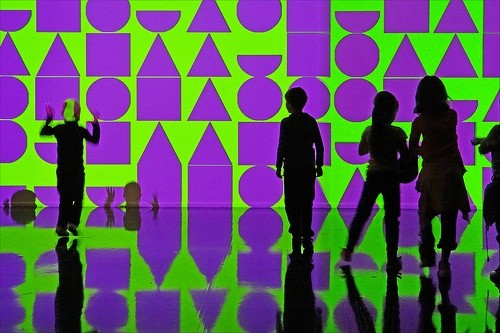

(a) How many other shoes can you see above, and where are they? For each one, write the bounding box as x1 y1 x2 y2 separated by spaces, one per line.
67 224 78 236
438 260 451 270
488 269 500 281
55 225 69 236
419 257 437 267
386 256 404 272
346 244 354 253
288 225 315 238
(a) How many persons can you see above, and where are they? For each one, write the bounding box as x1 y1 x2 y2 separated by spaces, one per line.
333 91 410 271
479 125 500 280
418 278 457 333
104 207 159 230
410 76 471 278
276 88 325 236
276 237 322 333
335 267 403 333
2 189 36 208
4 208 36 224
104 181 160 207
39 97 99 237
